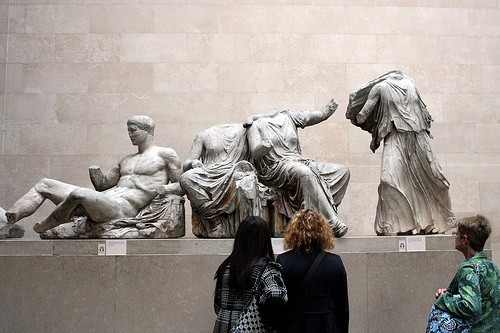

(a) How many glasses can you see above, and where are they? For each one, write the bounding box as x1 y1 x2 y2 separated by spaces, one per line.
452 231 466 238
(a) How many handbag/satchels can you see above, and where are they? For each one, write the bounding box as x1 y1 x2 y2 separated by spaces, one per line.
426 260 482 333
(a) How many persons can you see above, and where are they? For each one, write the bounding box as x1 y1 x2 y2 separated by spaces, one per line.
346 70 459 236
178 122 260 238
274 208 349 333
0 114 186 241
212 215 287 333
435 216 500 333
243 99 350 237
